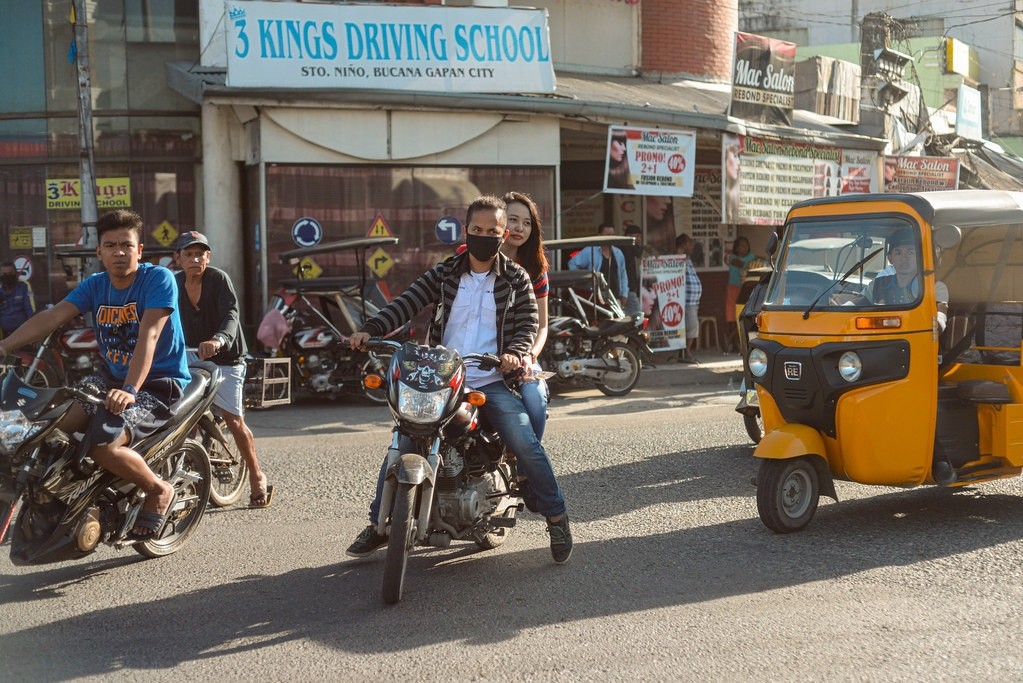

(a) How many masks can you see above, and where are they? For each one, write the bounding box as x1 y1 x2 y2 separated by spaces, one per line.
0 274 18 285
465 225 505 262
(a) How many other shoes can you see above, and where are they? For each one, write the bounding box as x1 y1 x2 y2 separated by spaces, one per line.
934 459 956 485
679 353 701 364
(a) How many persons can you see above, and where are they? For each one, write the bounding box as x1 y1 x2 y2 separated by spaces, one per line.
568 221 629 325
615 225 660 315
725 144 742 224
0 208 192 541
0 259 42 354
646 195 677 255
344 195 573 565
607 129 634 188
842 229 957 487
721 236 756 353
675 233 703 364
173 230 274 510
454 191 547 514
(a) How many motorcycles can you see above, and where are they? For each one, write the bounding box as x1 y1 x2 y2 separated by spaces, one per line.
336 332 527 603
243 284 404 412
0 297 254 557
537 296 657 397
736 190 1023 535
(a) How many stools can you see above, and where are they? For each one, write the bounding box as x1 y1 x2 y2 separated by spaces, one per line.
696 317 720 351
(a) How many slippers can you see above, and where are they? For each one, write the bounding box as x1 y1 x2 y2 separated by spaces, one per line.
248 484 274 509
129 491 177 541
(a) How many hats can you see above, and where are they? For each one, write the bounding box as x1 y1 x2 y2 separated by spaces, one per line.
176 231 211 252
888 228 915 253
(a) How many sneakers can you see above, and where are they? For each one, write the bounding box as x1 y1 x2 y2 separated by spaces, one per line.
543 512 573 564
516 479 539 512
345 520 391 557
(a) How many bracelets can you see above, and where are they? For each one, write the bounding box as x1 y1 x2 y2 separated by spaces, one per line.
122 384 138 398
529 352 537 364
212 335 226 347
